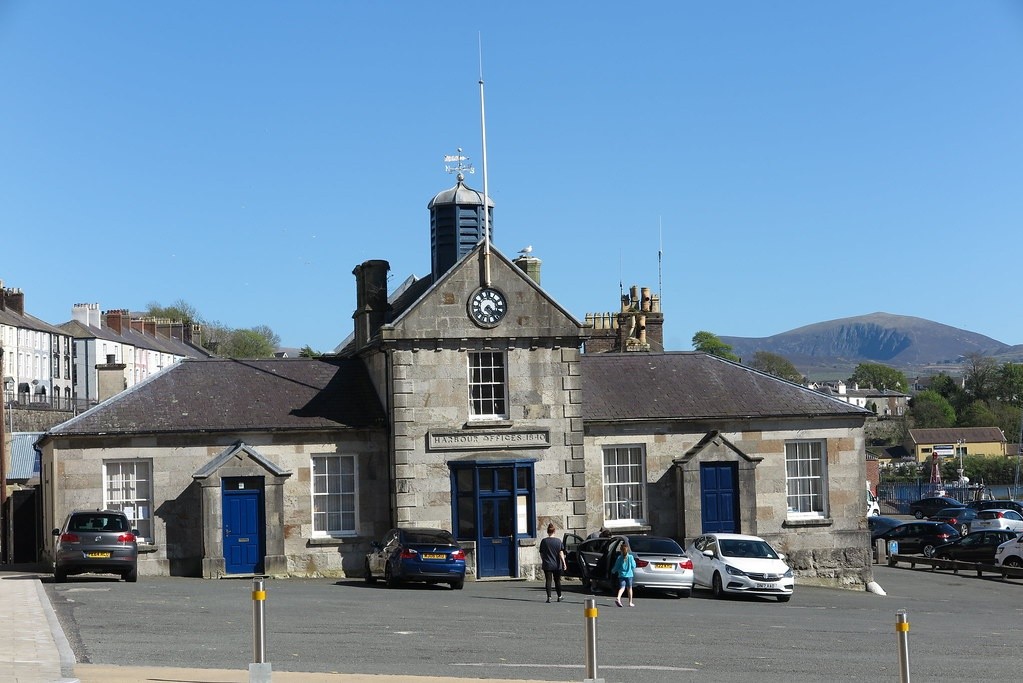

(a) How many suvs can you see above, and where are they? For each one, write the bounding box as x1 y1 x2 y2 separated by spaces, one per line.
994 533 1023 578
52 507 140 583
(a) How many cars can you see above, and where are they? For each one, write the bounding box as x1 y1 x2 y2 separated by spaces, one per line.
686 534 795 602
910 498 969 519
363 527 466 591
928 507 976 537
867 489 881 518
560 532 695 598
874 522 961 558
966 498 1023 516
972 509 1023 533
868 517 903 543
930 530 1017 569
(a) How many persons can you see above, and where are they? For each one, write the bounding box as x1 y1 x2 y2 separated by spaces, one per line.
612 543 636 607
539 523 567 603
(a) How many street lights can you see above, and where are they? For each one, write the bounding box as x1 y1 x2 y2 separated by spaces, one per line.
957 438 966 483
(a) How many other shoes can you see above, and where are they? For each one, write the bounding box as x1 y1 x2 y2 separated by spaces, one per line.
615 598 623 607
546 598 551 603
629 603 635 607
558 596 565 602
